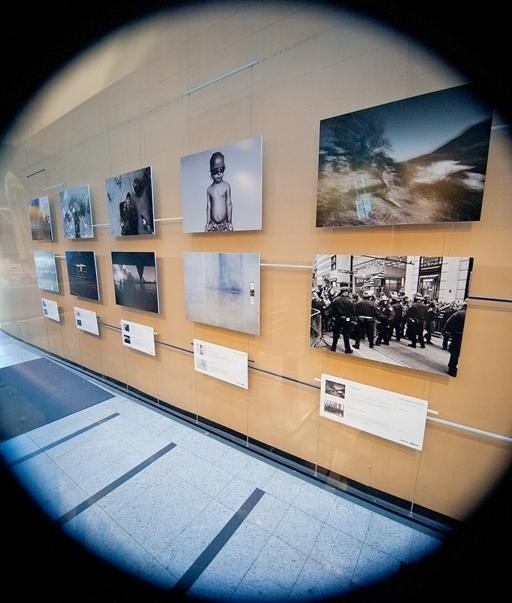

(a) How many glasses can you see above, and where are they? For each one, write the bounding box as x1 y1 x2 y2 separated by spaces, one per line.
213 166 225 175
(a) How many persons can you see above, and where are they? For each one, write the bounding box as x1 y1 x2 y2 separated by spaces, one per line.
204 152 234 232
311 279 468 376
120 192 135 230
